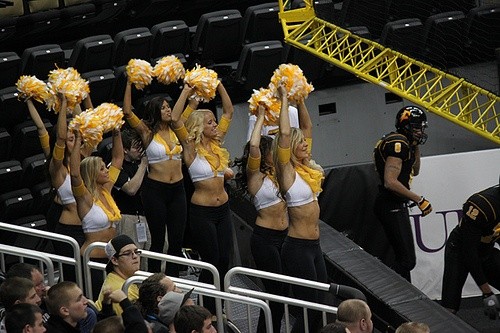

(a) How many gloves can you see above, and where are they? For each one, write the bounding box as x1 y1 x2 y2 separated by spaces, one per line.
482 292 497 316
418 196 433 217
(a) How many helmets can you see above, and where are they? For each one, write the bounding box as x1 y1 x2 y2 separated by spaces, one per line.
395 106 429 145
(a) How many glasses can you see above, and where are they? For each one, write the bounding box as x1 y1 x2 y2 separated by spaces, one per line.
117 250 142 258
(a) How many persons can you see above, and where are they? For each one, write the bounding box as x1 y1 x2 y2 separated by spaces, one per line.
373 105 432 284
438 182 499 320
170 67 234 328
105 127 150 274
335 299 373 333
319 322 352 333
395 321 430 333
122 58 201 282
70 120 123 301
0 233 195 333
25 87 87 296
277 80 330 333
174 305 218 333
236 101 290 333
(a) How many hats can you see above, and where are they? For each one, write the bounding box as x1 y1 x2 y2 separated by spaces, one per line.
104 234 136 274
157 288 194 325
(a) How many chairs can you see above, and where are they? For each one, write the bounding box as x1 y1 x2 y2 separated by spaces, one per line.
0 0 500 261
